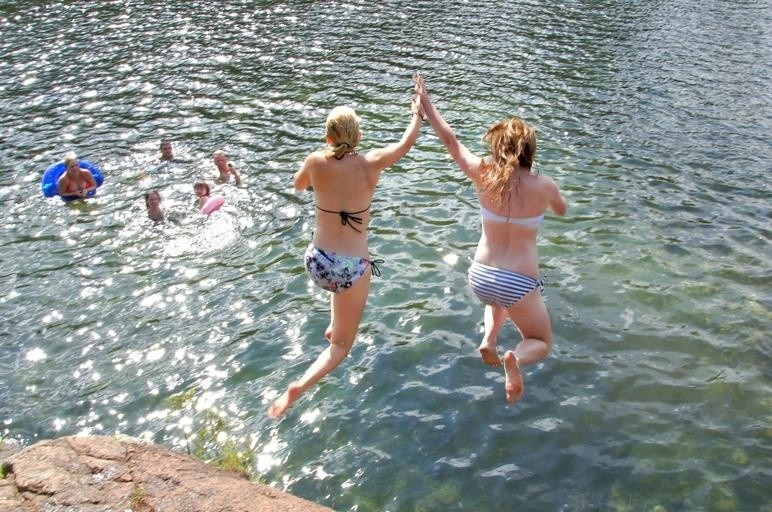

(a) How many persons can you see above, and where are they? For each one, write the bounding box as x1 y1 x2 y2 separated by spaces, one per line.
56 155 97 199
412 74 566 403
144 190 165 221
194 181 210 209
213 148 241 185
159 140 174 161
269 93 424 418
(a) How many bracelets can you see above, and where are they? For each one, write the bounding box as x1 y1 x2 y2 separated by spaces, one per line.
413 109 424 121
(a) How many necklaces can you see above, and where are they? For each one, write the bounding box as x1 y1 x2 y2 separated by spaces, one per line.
329 147 358 156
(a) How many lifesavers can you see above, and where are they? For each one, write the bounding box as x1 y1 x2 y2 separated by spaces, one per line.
201 195 224 214
42 160 104 200
